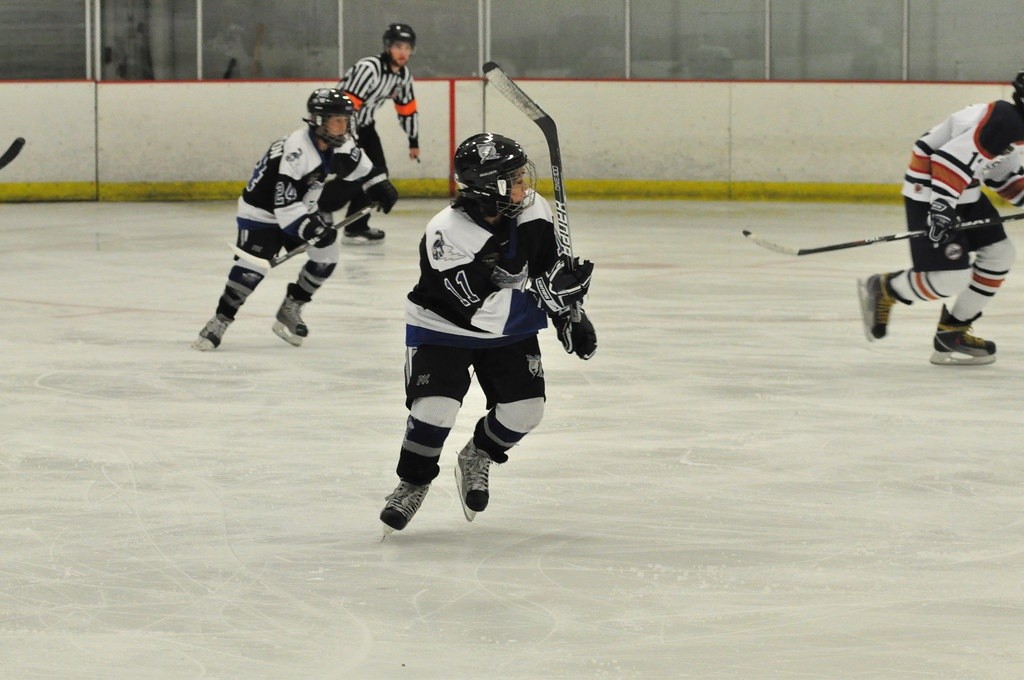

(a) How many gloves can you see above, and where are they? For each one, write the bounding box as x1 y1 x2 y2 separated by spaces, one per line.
928 199 963 244
551 305 597 359
534 256 593 317
298 213 336 249
366 179 400 214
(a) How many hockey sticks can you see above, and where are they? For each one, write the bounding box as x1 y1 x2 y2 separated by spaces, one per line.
482 61 585 351
268 197 385 267
0 137 27 174
742 212 1024 257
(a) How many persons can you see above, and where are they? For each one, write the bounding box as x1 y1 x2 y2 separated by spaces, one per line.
857 69 1024 366
192 87 399 350
379 131 597 542
319 23 421 244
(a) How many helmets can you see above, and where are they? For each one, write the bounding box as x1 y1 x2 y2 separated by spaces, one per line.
382 22 417 49
1012 71 1023 116
452 133 536 219
303 87 356 146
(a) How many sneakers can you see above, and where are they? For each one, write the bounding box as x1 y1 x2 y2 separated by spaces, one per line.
380 468 435 543
454 438 492 523
195 304 235 350
856 271 913 342
273 283 311 346
928 303 999 364
339 228 385 245
